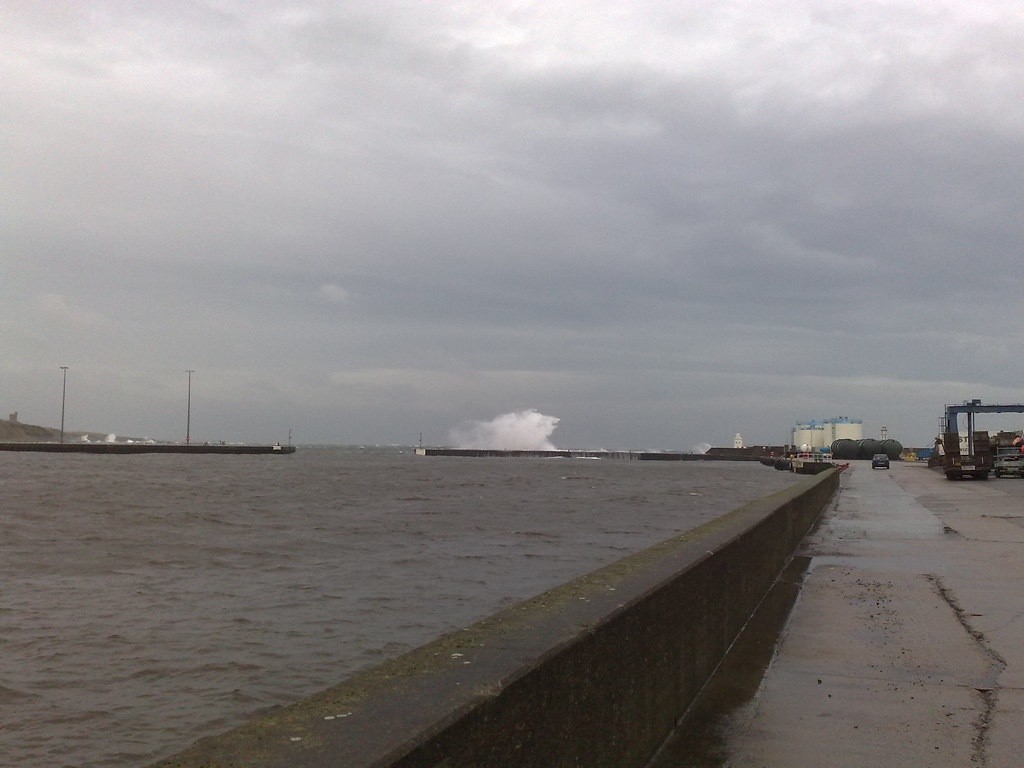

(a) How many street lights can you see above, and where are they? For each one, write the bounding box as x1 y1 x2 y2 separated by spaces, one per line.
59 366 69 444
184 370 196 446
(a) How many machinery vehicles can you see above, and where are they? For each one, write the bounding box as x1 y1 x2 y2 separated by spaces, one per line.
935 399 1024 480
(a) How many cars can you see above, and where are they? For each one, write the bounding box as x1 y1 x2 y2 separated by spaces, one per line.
871 454 890 469
